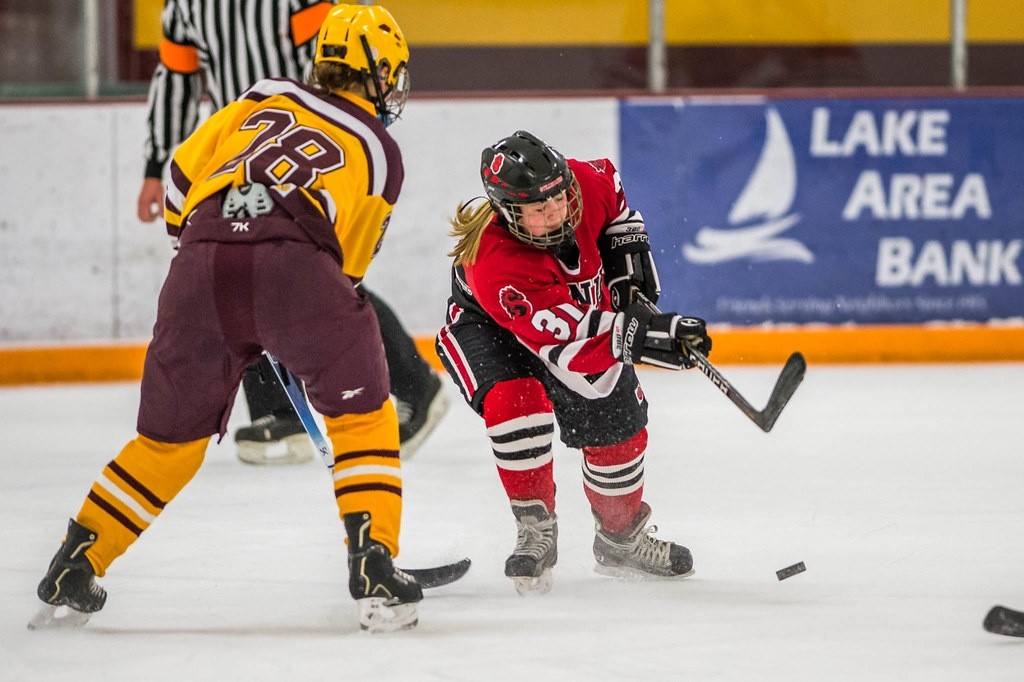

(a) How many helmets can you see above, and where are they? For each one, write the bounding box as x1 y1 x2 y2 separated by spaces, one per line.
480 130 583 249
313 4 410 126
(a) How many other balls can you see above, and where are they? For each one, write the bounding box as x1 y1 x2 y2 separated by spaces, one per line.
776 560 807 582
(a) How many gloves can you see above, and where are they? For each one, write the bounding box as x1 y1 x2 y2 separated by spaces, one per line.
603 232 660 312
621 302 710 371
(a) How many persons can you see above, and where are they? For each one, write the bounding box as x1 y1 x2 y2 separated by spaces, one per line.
435 130 712 595
27 3 424 632
138 0 449 464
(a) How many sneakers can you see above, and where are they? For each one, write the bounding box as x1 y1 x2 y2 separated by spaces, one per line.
504 482 559 598
342 510 424 635
590 501 694 581
27 518 107 630
236 412 315 465
396 372 447 459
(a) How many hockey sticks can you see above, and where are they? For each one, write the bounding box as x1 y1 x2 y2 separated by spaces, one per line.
263 353 473 589
629 286 806 434
